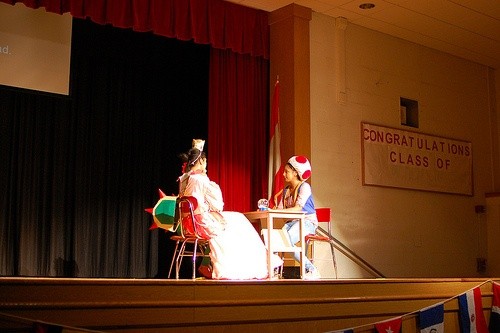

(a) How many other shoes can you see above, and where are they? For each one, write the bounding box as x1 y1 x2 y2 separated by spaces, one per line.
303 267 321 280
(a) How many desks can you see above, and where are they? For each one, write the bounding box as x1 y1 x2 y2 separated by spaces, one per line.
244 212 307 280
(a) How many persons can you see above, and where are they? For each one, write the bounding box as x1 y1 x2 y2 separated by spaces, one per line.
268 155 323 279
177 139 284 280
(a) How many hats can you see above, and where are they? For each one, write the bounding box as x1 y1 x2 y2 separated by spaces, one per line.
288 155 312 181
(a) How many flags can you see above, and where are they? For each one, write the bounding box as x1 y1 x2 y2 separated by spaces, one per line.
374 318 402 333
458 287 488 333
333 329 353 333
419 304 445 333
488 280 500 333
267 75 286 211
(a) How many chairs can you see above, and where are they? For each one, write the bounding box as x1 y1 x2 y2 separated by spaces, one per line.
279 207 338 278
167 196 212 280
